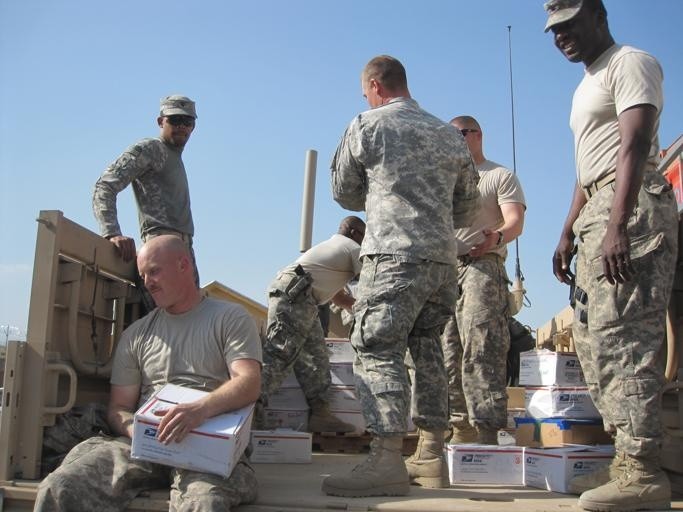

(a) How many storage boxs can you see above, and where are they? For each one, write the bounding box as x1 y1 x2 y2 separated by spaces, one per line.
265 338 371 434
515 350 618 498
443 442 523 487
131 383 252 479
250 430 312 463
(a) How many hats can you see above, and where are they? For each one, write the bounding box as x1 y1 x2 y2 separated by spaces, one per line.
160 96 197 118
543 1 584 33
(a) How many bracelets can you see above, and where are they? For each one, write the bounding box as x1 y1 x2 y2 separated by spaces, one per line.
496 230 503 245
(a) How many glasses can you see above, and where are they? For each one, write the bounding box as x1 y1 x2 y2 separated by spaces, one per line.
461 129 478 137
166 117 194 126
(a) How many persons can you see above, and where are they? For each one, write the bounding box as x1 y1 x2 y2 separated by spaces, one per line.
251 216 366 434
32 235 263 512
92 96 198 312
445 115 525 444
543 0 678 512
319 54 481 499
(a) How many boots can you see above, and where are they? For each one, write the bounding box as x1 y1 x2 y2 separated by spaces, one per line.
579 457 671 510
404 429 450 488
567 452 628 494
322 436 410 497
307 399 355 432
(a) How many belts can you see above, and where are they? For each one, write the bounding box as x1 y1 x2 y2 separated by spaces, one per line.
459 253 504 266
582 165 653 199
